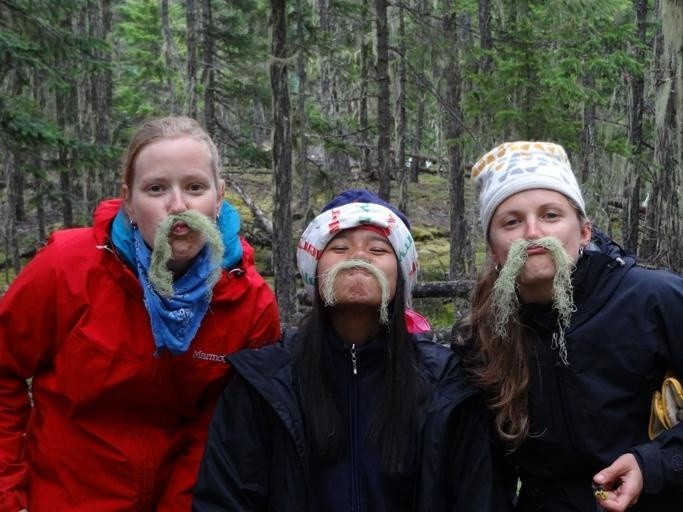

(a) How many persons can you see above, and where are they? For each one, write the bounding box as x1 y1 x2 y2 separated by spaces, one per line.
193 187 497 511
452 138 682 510
0 115 284 511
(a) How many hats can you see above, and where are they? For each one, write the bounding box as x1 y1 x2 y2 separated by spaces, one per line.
471 141 587 242
295 189 419 306
